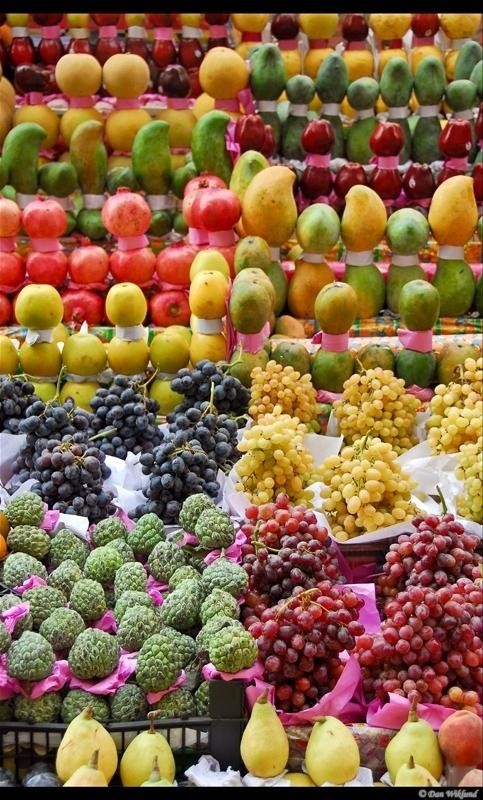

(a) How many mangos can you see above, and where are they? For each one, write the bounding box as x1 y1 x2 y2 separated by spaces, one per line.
249 40 482 163
0 109 233 239
229 150 483 318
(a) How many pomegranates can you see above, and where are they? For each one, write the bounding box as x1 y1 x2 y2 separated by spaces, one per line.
1 170 242 327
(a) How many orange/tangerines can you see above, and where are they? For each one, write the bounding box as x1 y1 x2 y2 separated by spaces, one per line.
229 235 483 392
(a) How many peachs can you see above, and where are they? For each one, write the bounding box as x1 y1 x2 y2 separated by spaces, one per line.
437 710 483 768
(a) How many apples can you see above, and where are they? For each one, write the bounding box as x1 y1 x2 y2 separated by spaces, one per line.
236 101 483 218
1 248 229 416
1 13 229 99
272 14 441 39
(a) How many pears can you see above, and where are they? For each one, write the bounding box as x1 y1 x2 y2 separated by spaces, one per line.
55 687 444 787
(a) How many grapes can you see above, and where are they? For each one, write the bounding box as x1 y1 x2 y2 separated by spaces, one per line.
0 358 252 527
242 492 483 712
235 356 483 542
